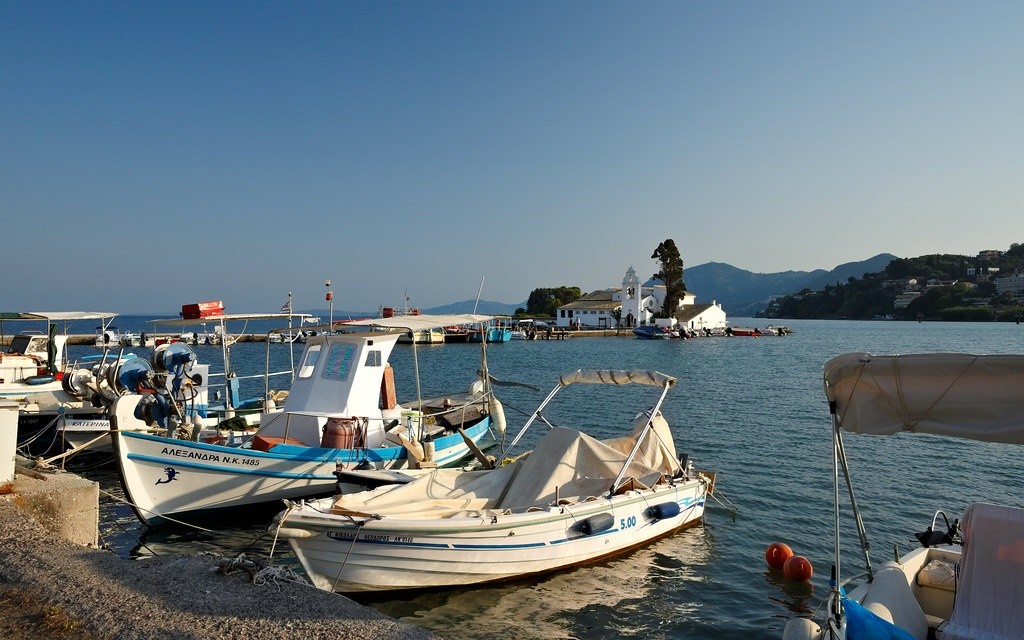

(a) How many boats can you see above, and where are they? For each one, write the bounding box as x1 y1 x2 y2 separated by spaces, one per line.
296 330 326 343
632 325 671 340
94 326 119 347
208 326 235 345
731 327 763 336
821 351 1024 640
56 314 313 451
180 331 206 345
331 314 538 344
0 311 139 439
266 335 292 343
109 313 496 527
269 371 716 593
124 333 141 346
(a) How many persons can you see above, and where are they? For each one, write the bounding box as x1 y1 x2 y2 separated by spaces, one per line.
569 317 572 325
575 316 580 331
